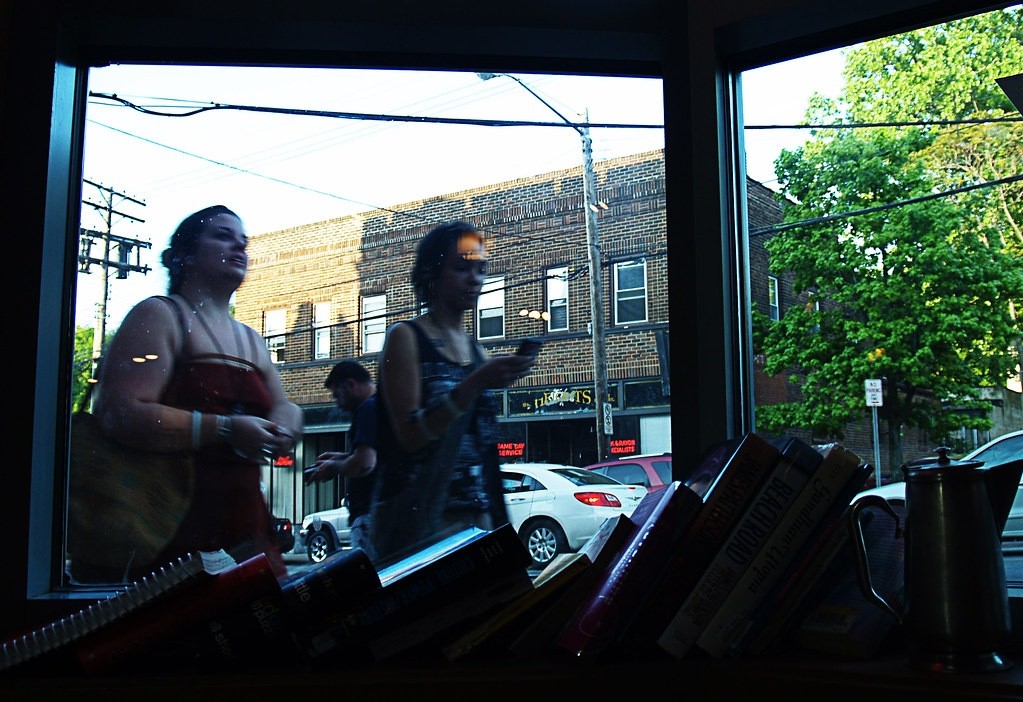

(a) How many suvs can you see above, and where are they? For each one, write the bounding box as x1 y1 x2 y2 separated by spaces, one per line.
577 452 672 493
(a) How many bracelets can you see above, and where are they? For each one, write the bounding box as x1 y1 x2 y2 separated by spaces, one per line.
216 415 231 446
191 410 202 450
443 393 468 420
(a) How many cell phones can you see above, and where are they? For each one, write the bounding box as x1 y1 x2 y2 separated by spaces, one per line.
517 338 544 357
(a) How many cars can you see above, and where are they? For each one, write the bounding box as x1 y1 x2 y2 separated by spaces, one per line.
469 464 648 569
850 430 1023 605
275 517 296 555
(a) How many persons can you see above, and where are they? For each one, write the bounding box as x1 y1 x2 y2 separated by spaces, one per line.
306 361 375 571
78 204 303 582
376 222 538 553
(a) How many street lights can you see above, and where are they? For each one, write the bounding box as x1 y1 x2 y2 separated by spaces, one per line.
479 70 613 465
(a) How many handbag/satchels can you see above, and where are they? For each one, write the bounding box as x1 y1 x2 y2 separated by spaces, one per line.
64 297 194 568
